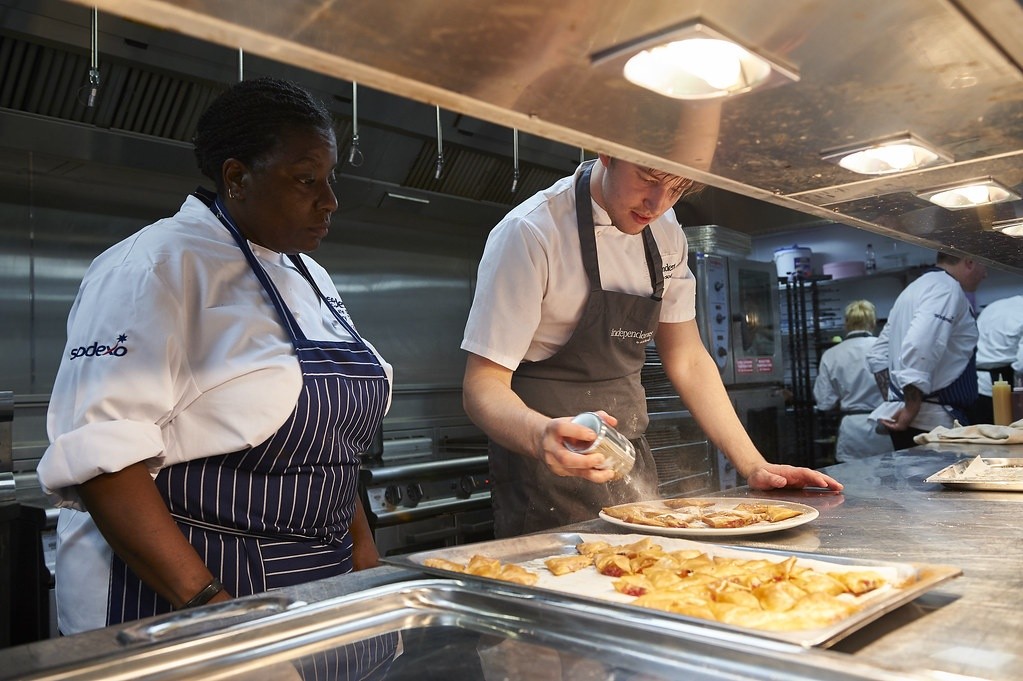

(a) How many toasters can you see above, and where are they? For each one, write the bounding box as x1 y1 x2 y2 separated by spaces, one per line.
865 243 876 274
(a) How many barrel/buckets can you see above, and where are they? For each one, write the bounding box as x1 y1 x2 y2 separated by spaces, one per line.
772 243 813 287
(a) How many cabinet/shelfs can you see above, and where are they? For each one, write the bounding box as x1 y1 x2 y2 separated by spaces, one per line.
778 264 928 465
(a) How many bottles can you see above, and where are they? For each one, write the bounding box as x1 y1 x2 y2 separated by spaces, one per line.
992 374 1023 426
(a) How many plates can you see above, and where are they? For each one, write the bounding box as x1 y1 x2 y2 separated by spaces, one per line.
598 497 819 535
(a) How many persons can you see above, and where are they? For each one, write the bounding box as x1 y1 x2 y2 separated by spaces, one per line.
811 298 898 465
973 294 1023 425
460 155 843 540
870 249 980 453
35 74 394 636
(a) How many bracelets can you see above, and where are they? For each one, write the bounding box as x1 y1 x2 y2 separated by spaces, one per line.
186 577 223 610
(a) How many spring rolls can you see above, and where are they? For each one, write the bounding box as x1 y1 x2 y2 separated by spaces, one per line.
423 537 886 635
600 497 805 529
